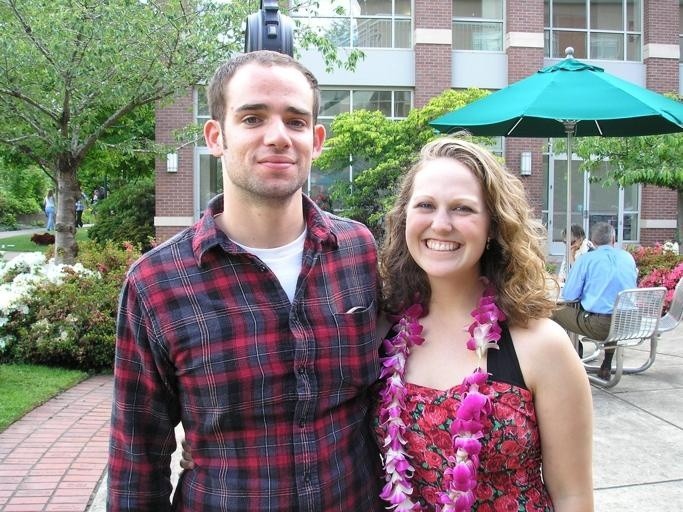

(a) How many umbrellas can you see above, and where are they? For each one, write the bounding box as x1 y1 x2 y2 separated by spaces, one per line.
427 47 683 280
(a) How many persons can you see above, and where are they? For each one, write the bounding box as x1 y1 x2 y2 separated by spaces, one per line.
180 137 594 512
548 222 638 381
108 50 387 512
43 187 101 231
557 224 595 287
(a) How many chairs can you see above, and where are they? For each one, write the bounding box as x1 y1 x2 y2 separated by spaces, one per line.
582 275 683 391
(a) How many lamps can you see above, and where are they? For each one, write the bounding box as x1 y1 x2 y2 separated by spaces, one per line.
519 151 532 178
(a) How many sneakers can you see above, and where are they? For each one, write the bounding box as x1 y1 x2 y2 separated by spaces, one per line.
597 369 611 379
